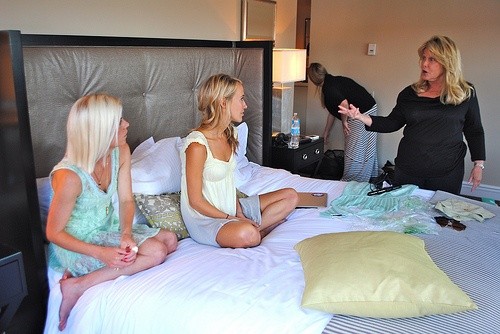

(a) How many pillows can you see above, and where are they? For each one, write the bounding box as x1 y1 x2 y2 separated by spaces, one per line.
293 231 478 319
35 122 251 240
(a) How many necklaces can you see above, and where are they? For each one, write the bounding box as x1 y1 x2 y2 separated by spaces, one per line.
94 167 104 185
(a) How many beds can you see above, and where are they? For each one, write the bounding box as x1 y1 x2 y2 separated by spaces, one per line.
42 163 500 334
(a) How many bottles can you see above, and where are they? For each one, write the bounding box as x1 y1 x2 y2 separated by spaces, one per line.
290 113 300 146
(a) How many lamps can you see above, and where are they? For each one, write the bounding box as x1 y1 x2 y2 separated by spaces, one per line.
272 48 307 90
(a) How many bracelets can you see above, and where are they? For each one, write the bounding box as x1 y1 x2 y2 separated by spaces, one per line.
474 163 484 169
226 214 229 219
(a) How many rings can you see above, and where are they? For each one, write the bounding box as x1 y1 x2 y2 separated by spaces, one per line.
131 246 138 253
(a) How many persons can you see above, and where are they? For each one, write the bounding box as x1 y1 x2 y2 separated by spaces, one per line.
45 94 177 331
179 73 298 248
336 34 485 195
308 62 379 182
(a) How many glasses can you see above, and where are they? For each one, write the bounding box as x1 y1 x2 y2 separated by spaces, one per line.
434 216 466 231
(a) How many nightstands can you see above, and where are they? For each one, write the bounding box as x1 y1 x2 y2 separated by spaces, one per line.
272 135 324 174
0 243 29 334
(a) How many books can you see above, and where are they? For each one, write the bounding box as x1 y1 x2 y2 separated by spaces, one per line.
297 192 328 208
